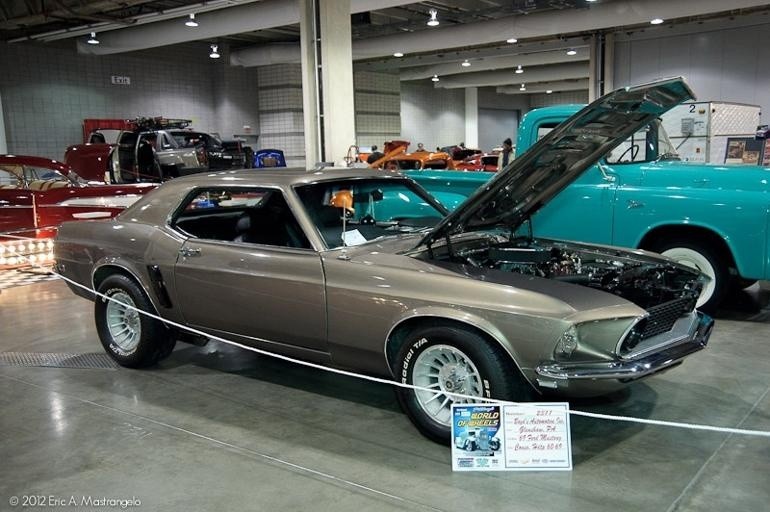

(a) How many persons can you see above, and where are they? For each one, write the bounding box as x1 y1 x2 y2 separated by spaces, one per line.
497 138 516 172
368 145 385 164
416 143 425 152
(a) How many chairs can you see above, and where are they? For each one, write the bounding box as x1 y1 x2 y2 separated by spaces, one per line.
231 206 284 245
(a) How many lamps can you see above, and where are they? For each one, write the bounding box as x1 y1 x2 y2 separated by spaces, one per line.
87 31 100 45
425 7 440 27
514 65 524 74
431 74 439 82
186 13 199 28
519 83 527 91
207 44 221 59
